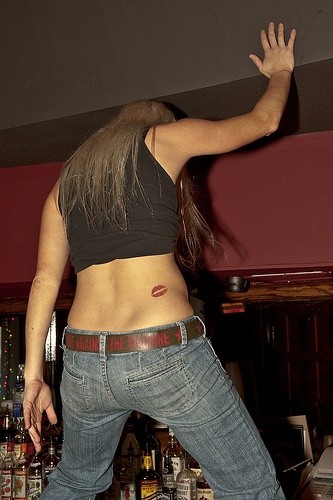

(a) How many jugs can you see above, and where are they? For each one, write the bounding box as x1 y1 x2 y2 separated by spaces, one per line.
226 276 247 292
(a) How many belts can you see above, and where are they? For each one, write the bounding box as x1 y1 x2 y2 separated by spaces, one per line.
62 319 204 353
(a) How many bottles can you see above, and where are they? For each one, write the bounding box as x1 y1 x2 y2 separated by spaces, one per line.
0 366 65 500
96 412 215 500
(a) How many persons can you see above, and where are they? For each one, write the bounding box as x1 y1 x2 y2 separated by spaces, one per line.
22 21 297 500
174 240 246 402
